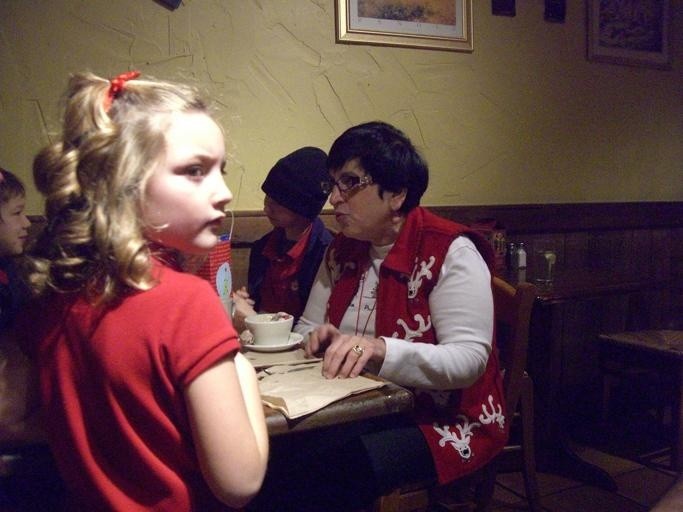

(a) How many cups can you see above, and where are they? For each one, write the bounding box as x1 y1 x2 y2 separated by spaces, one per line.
246 312 294 346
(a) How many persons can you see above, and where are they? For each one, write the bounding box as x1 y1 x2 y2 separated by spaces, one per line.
0 168 32 318
0 68 271 510
234 145 334 322
293 120 509 512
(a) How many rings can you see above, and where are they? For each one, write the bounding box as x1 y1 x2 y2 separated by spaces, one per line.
351 343 364 356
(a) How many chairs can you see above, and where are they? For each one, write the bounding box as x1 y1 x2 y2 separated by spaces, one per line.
370 260 554 510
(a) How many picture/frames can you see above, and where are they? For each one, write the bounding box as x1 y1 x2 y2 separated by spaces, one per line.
585 1 674 68
333 1 474 56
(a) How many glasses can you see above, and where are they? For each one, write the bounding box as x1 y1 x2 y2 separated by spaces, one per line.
320 173 374 194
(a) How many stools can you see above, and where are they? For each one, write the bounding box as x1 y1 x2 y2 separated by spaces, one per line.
599 319 683 471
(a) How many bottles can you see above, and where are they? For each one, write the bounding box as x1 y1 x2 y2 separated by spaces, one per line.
486 227 530 273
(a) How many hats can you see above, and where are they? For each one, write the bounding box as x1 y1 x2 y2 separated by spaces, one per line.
261 147 332 217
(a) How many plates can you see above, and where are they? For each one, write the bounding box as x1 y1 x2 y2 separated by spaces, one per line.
239 331 304 352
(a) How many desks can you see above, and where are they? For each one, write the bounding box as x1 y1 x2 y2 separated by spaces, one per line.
488 265 678 490
258 342 413 438
